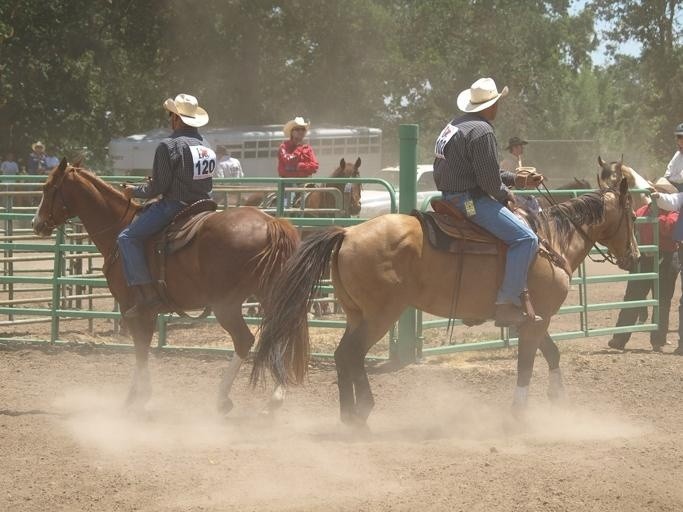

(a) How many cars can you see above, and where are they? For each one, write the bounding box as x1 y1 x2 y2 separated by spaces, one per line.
350 163 442 219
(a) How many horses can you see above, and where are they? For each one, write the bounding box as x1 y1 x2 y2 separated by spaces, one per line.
248 176 642 432
597 154 683 355
30 154 311 415
235 156 364 319
534 171 593 208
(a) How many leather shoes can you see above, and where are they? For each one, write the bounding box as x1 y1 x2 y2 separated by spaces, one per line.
495 303 543 327
125 299 161 319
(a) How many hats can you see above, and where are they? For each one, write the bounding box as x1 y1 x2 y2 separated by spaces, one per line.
505 137 529 150
456 75 509 114
31 141 45 152
645 176 680 194
216 145 230 155
162 93 210 127
674 122 683 136
284 117 311 138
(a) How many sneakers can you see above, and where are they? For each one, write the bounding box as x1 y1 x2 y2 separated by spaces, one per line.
608 339 625 350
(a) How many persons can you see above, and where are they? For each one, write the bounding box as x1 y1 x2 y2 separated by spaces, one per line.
606 177 681 353
650 190 683 356
274 117 319 207
0 141 59 206
210 144 242 205
655 123 683 196
116 93 218 320
433 78 543 326
500 136 541 214
